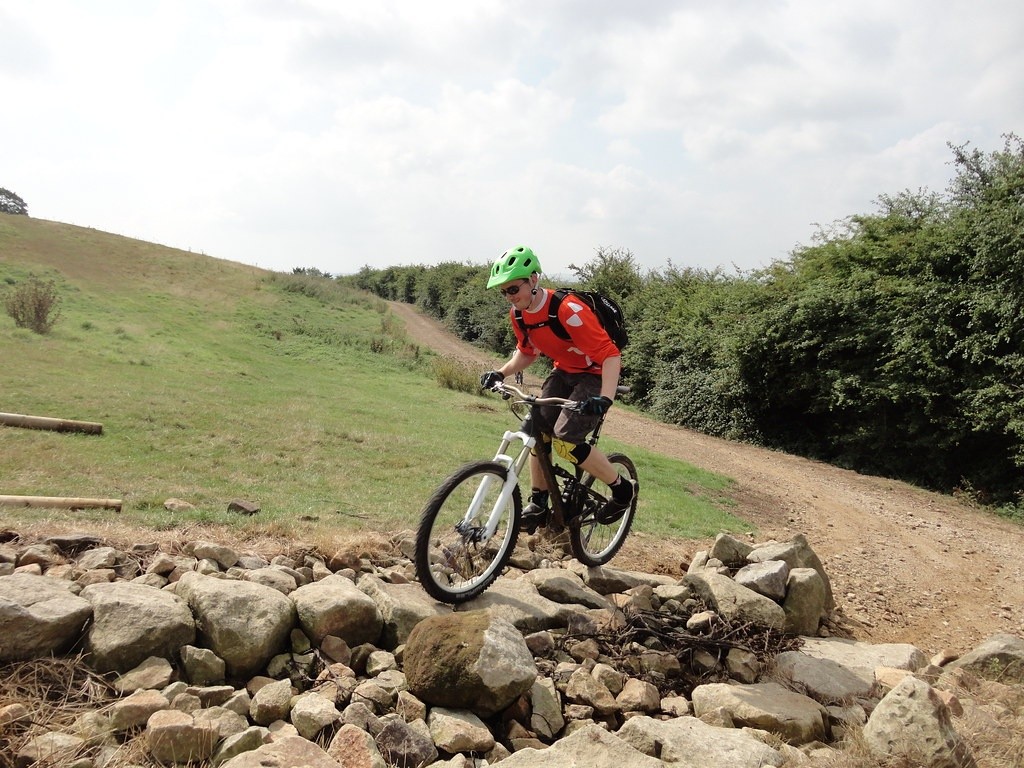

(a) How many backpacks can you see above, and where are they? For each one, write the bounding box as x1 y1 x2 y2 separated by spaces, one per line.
549 285 629 367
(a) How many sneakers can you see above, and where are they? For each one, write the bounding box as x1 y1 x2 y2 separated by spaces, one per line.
596 479 640 525
519 488 551 529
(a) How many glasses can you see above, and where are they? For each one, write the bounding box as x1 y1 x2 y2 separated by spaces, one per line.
500 279 529 297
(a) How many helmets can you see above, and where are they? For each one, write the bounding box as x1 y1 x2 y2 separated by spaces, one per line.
486 245 543 311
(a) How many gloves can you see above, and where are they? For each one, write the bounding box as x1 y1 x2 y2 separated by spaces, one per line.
578 396 613 417
479 370 505 390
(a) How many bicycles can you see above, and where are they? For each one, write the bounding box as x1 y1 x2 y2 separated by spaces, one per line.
413 369 640 606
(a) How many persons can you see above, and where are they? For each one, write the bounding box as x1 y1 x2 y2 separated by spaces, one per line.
481 245 640 525
